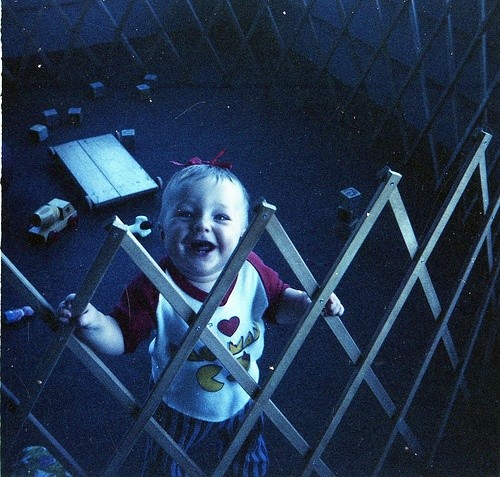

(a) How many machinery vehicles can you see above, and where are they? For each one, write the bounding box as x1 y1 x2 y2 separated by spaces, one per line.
27 197 81 246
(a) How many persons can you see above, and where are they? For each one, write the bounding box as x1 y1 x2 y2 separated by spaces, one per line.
58 148 344 477
